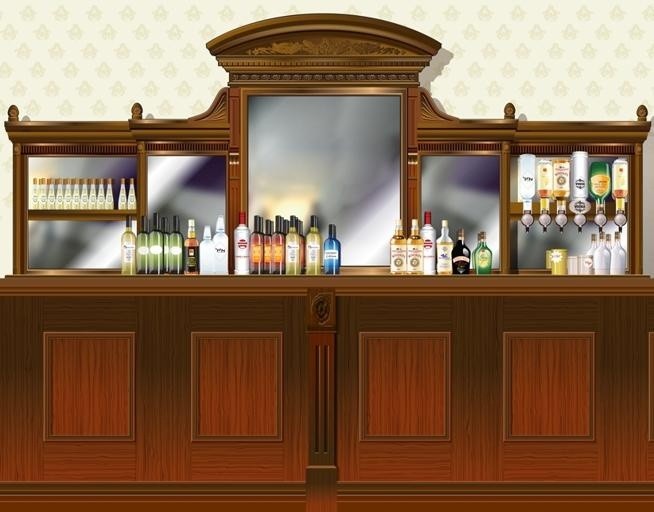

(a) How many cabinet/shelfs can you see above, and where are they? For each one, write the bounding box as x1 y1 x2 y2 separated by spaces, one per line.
0 13 653 511
